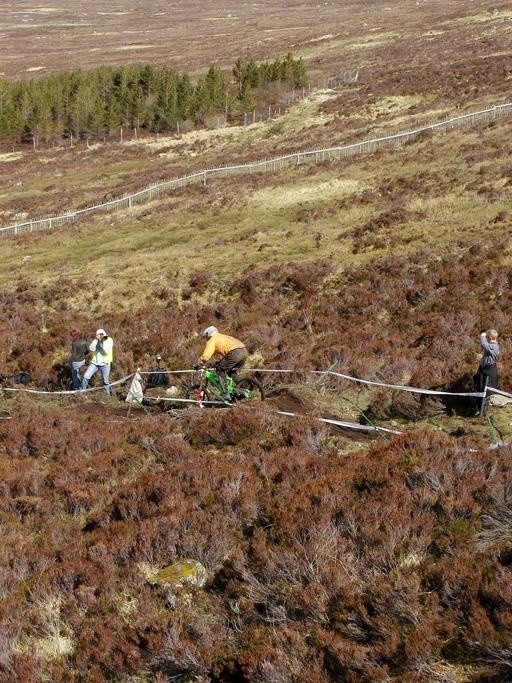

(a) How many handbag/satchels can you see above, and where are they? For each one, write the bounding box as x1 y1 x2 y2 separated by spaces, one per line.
473 372 480 389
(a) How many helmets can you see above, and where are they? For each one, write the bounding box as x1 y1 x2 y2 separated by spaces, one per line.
201 326 218 338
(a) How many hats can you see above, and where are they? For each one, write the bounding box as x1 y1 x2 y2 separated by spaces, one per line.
95 329 106 336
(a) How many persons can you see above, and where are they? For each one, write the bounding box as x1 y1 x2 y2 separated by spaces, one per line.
472 324 499 415
193 324 250 401
69 328 91 390
78 327 115 394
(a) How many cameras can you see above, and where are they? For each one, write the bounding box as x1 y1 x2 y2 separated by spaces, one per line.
99 334 107 339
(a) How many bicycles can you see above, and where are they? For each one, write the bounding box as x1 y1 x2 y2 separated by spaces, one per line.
186 361 265 408
